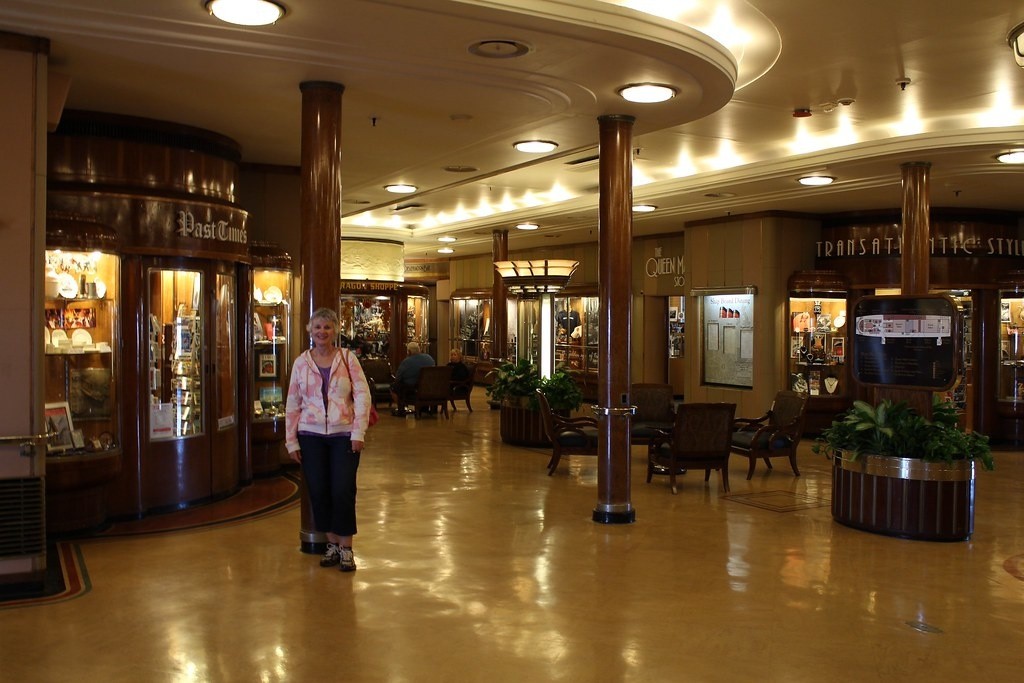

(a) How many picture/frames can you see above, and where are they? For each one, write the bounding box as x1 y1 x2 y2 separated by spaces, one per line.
256 347 281 381
68 367 114 422
259 386 286 412
43 401 76 454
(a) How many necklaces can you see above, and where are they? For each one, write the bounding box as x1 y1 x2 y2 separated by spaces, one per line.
827 378 836 388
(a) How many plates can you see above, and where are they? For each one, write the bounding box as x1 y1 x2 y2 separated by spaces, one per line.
44 327 50 348
71 328 93 347
90 280 107 298
56 273 78 298
51 329 68 348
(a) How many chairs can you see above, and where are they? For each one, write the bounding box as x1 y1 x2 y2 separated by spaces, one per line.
531 383 810 498
357 353 479 420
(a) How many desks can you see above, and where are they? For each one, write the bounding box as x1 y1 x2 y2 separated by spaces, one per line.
45 347 112 424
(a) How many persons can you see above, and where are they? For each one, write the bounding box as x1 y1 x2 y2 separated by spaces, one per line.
388 342 436 419
557 302 582 337
445 347 470 395
282 309 372 573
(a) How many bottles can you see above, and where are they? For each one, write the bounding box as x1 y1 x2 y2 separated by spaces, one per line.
45 263 60 297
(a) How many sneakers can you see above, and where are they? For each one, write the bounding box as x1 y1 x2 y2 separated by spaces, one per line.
338 547 356 570
320 543 343 567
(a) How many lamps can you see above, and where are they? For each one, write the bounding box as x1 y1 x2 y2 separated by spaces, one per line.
995 150 1024 165
797 175 835 186
633 204 659 214
513 139 559 153
383 184 418 194
615 82 679 103
1006 21 1024 70
515 223 541 230
205 0 286 26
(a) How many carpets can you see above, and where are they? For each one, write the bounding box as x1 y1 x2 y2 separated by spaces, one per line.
718 490 833 513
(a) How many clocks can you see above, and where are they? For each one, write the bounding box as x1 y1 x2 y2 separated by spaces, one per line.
98 430 115 450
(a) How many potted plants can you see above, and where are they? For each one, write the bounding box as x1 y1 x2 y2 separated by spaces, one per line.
484 358 584 449
805 392 998 544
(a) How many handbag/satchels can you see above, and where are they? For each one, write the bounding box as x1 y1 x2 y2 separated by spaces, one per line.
338 346 379 426
